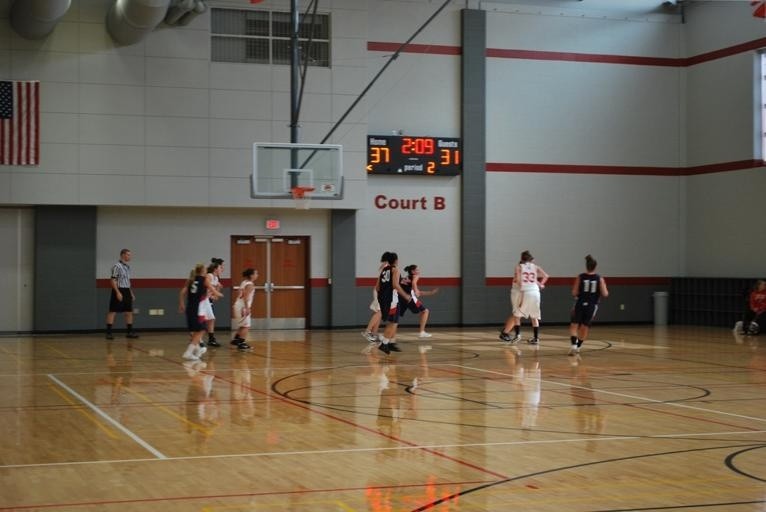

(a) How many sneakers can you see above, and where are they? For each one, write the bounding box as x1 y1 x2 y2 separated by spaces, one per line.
182 333 251 360
511 334 520 344
360 328 402 354
527 337 539 344
105 333 112 339
500 334 510 341
125 332 139 338
575 346 580 353
418 330 432 338
567 344 577 356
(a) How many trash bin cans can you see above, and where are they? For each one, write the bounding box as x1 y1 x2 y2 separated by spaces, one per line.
651 291 671 325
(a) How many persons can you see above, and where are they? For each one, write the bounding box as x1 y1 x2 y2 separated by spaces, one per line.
362 250 440 352
366 347 430 463
500 250 610 354
106 246 140 343
180 257 258 364
104 345 140 429
511 353 611 455
172 366 261 451
739 279 765 336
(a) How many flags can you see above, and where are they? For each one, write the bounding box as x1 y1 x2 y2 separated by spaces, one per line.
2 81 41 166
